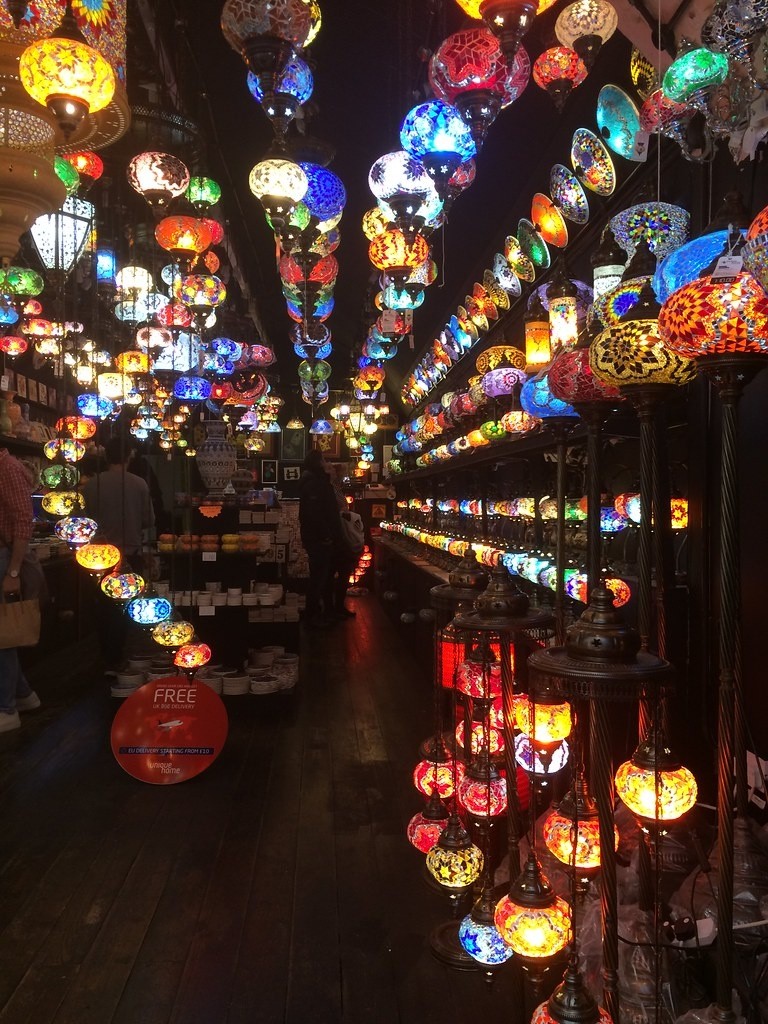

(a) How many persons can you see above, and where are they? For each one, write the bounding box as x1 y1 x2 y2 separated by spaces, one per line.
0 447 41 732
72 444 157 676
302 450 359 630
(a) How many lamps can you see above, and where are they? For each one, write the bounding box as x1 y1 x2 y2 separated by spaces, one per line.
0 0 768 1024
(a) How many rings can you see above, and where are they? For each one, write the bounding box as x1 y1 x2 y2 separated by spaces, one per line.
8 593 14 598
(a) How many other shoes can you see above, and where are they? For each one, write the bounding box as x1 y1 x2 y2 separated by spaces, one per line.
105 669 119 679
304 618 341 631
336 608 356 616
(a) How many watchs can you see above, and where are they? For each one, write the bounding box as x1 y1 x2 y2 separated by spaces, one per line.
5 569 21 578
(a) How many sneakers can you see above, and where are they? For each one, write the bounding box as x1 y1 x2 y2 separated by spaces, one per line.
0 709 21 733
15 692 41 712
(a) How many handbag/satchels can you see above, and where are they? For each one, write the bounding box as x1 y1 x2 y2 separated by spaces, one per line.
0 591 41 650
